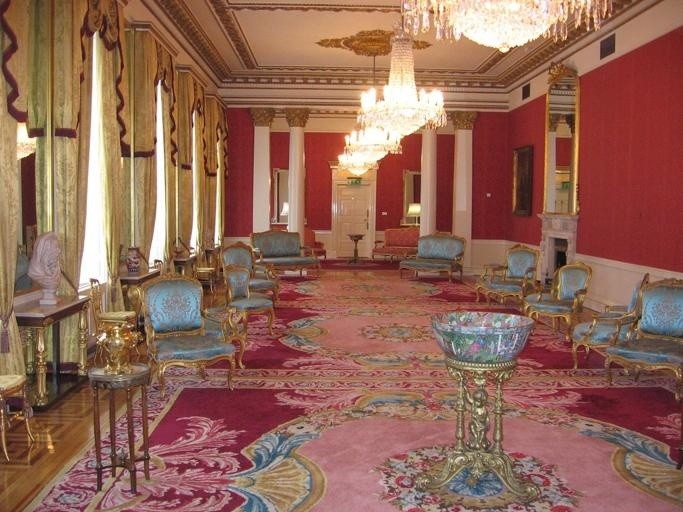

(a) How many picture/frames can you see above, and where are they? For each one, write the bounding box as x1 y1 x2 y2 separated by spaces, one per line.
511 144 531 216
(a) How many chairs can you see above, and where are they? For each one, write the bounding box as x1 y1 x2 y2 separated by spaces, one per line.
522 262 592 343
571 273 650 384
0 374 31 462
223 264 275 343
154 259 164 276
219 242 278 307
604 278 683 403
476 244 540 309
302 227 327 265
89 278 142 365
138 273 235 398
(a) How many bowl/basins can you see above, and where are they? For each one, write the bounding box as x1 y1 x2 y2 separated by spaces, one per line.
348 233 365 240
430 310 538 365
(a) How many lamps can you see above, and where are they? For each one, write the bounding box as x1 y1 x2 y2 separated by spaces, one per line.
398 0 615 55
407 203 422 226
281 201 291 230
338 37 448 177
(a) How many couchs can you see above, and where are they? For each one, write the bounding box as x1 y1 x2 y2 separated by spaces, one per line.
371 228 451 262
249 229 322 281
399 234 465 283
